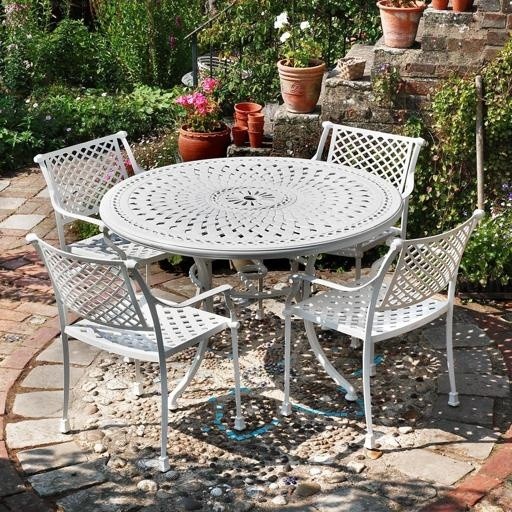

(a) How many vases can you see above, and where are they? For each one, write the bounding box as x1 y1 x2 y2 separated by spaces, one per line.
276 57 325 113
376 0 474 48
177 123 230 164
171 77 227 130
272 11 316 68
232 100 265 149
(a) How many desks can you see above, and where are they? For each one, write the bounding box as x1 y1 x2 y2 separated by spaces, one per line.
99 156 403 411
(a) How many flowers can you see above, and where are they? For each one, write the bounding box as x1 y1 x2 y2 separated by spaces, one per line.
272 11 316 68
171 77 227 130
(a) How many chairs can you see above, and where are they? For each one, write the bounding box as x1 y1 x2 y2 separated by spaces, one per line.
27 234 246 471
282 209 486 449
288 120 424 307
33 131 174 310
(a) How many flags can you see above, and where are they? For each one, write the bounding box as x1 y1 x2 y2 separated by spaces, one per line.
232 100 265 149
177 123 230 164
376 0 474 48
276 57 325 113
